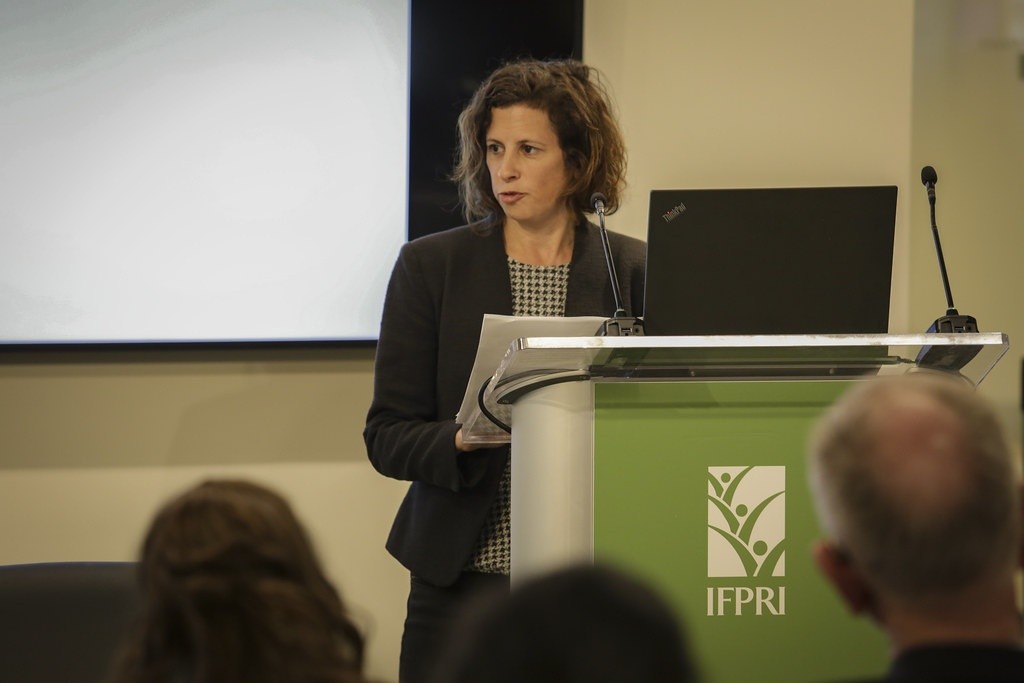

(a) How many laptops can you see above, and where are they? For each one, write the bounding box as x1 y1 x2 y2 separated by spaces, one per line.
642 185 897 377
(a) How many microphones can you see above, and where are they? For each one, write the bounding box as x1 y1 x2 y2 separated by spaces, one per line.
919 164 984 372
590 194 649 377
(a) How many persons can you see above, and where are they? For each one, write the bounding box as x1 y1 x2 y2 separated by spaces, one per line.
106 478 363 683
362 57 705 683
799 368 1024 683
422 563 703 683
